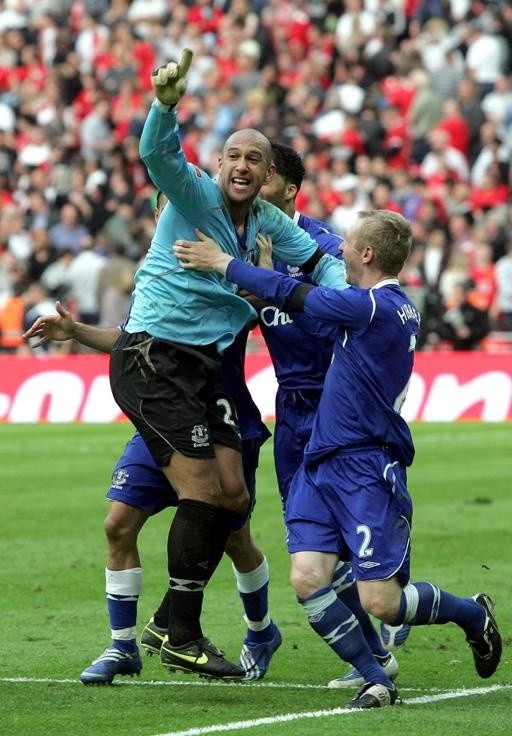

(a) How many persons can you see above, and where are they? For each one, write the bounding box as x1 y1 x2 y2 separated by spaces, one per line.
241 143 414 689
172 207 501 711
0 1 512 355
109 47 349 683
21 190 282 684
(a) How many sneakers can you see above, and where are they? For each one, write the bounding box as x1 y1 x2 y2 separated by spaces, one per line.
78 646 144 688
464 592 502 679
236 620 283 682
379 616 412 652
343 680 405 710
327 650 401 689
139 615 248 683
